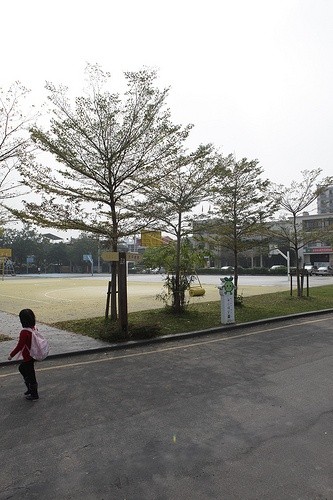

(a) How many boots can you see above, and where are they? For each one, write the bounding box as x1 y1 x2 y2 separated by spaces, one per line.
23 381 40 400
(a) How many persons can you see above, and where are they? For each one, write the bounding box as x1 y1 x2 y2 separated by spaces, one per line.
8 309 40 402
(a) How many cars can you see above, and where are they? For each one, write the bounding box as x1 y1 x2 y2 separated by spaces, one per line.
316 266 333 276
141 266 166 274
220 265 235 271
300 266 318 276
268 264 286 270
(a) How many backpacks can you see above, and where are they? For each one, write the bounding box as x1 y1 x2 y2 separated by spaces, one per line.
17 328 49 361
